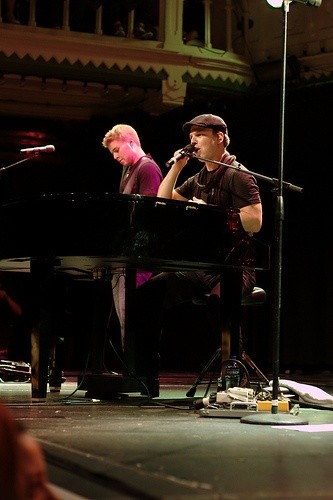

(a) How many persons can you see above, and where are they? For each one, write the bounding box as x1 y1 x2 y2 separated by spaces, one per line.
123 114 262 378
104 124 162 339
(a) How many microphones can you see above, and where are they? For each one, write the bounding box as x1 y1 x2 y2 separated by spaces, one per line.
166 144 195 167
20 145 55 153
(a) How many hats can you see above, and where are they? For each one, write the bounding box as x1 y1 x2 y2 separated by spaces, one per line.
183 113 228 133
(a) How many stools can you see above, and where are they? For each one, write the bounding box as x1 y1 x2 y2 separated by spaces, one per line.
186 286 271 397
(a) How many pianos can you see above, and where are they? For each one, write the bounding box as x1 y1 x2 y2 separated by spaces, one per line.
0 189 244 406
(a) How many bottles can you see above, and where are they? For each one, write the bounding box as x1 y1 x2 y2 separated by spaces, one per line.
225 356 240 389
